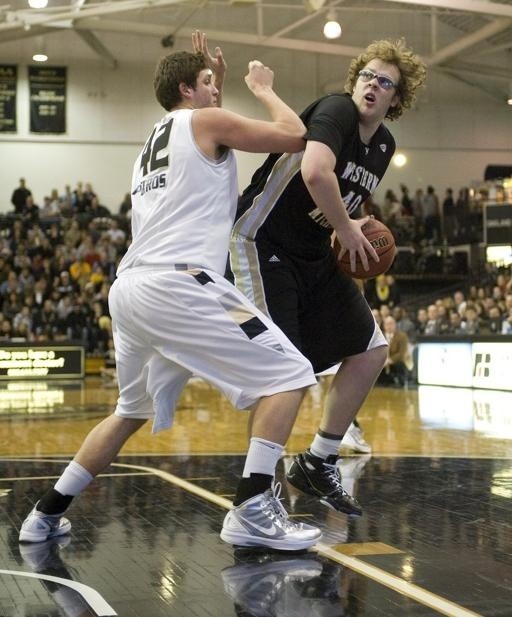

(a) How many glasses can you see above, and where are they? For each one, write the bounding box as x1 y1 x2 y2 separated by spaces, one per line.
358 68 400 91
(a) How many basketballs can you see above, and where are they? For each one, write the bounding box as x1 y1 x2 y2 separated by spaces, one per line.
333 218 395 279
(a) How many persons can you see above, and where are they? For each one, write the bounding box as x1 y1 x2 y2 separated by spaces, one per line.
17 549 323 617
1 178 132 379
280 451 375 545
350 177 512 388
319 361 375 456
225 37 425 521
15 22 324 549
286 538 365 616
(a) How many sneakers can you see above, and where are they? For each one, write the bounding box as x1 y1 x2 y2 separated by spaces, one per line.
19 537 72 571
286 447 363 518
341 419 373 454
219 482 323 551
18 500 72 543
221 559 325 617
